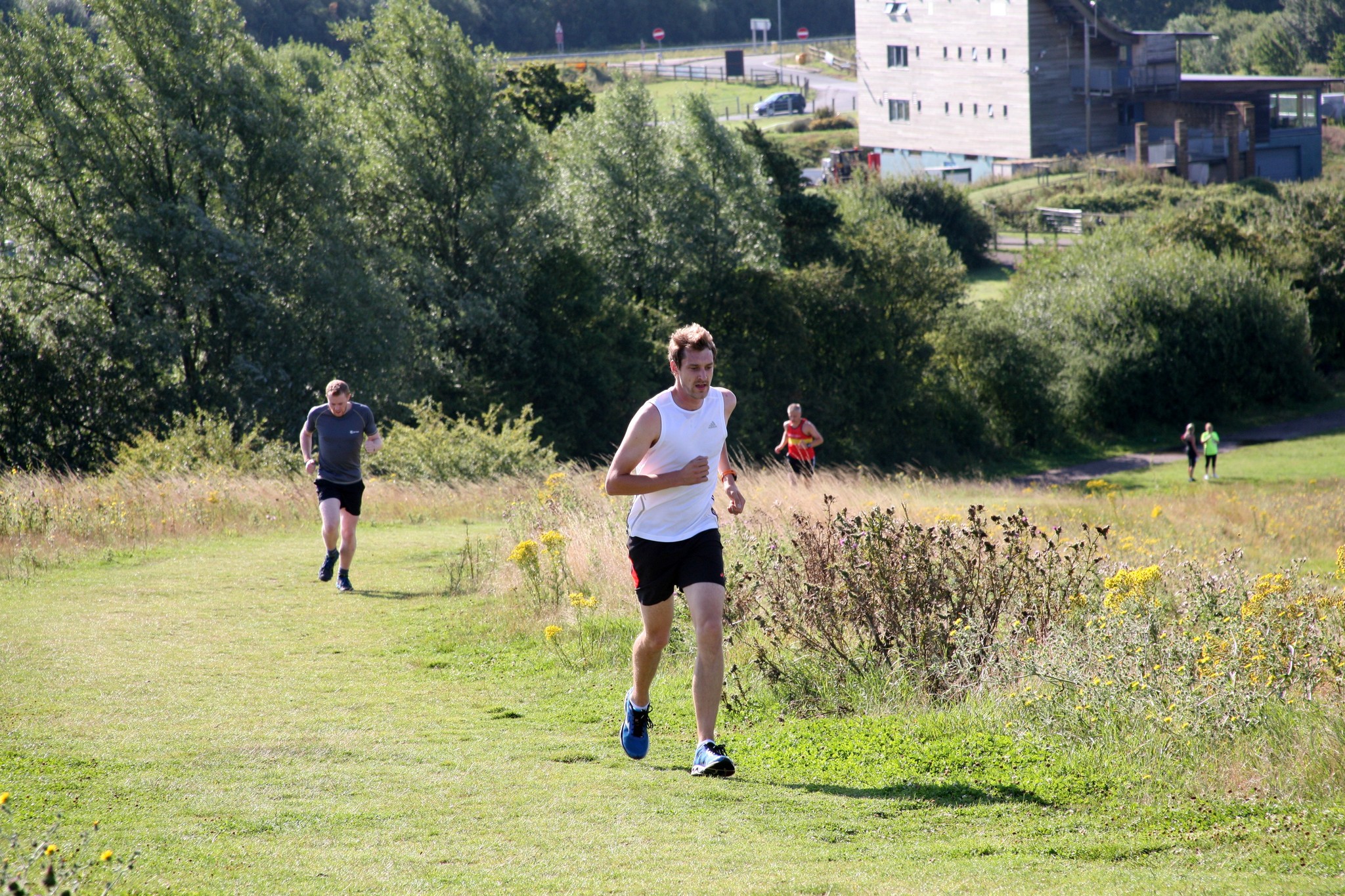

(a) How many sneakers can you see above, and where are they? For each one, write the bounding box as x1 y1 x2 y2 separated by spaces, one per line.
691 742 736 777
619 687 654 760
318 550 340 582
336 577 354 592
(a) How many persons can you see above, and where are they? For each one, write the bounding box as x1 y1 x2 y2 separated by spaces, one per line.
1200 423 1219 480
299 379 383 590
1181 423 1199 481
606 323 746 777
774 403 823 488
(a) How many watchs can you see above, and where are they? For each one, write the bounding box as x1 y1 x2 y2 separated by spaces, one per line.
720 470 737 483
806 443 811 448
